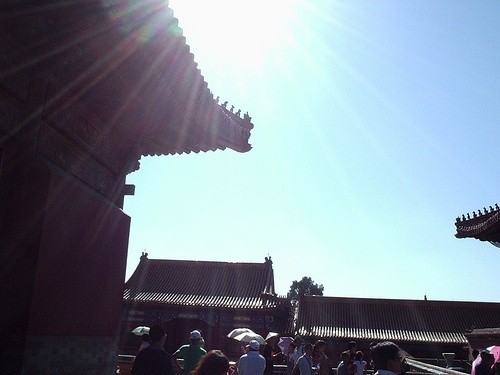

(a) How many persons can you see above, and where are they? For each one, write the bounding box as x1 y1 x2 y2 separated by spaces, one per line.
202 352 229 375
237 340 266 375
472 349 500 375
131 325 208 375
284 336 402 375
262 332 278 375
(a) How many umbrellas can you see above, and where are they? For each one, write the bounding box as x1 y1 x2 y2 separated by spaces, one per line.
471 345 500 375
233 332 267 346
227 328 255 339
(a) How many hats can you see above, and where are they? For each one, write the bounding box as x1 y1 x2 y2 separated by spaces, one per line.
264 332 278 342
293 335 305 343
190 330 201 340
246 340 259 350
